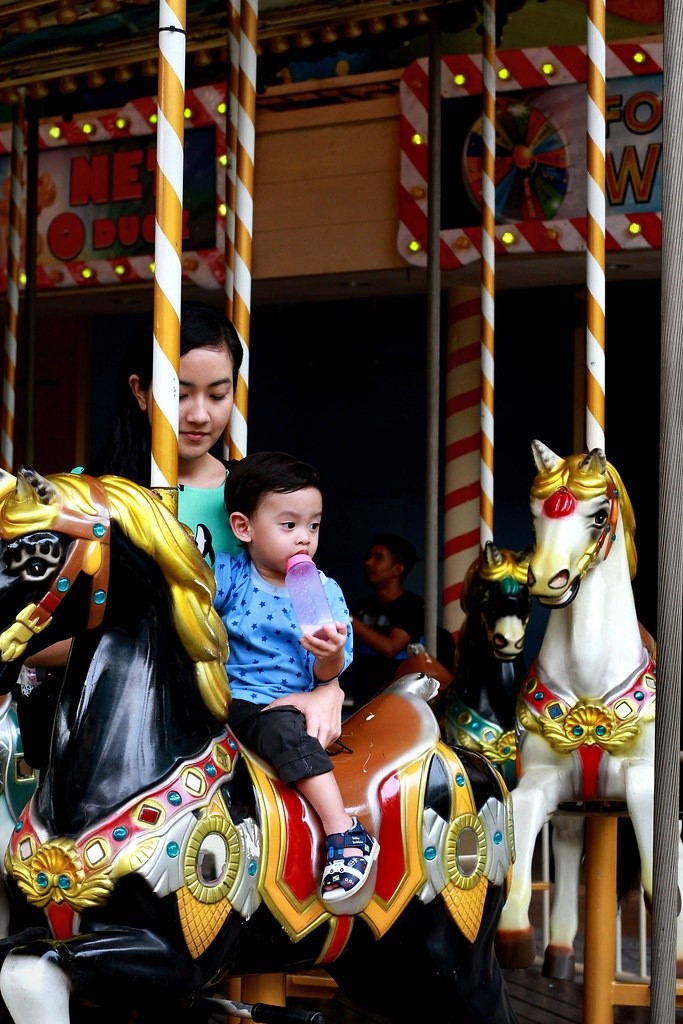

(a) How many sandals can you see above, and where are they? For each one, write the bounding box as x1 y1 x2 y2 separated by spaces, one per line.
316 817 381 903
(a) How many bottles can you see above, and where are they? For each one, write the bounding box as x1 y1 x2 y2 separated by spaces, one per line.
286 555 338 642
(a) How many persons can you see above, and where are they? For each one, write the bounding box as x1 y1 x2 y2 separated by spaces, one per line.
206 450 380 904
26 300 345 747
351 534 427 711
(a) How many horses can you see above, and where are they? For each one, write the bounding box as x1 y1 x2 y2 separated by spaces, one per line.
457 440 683 986
0 461 514 1024
